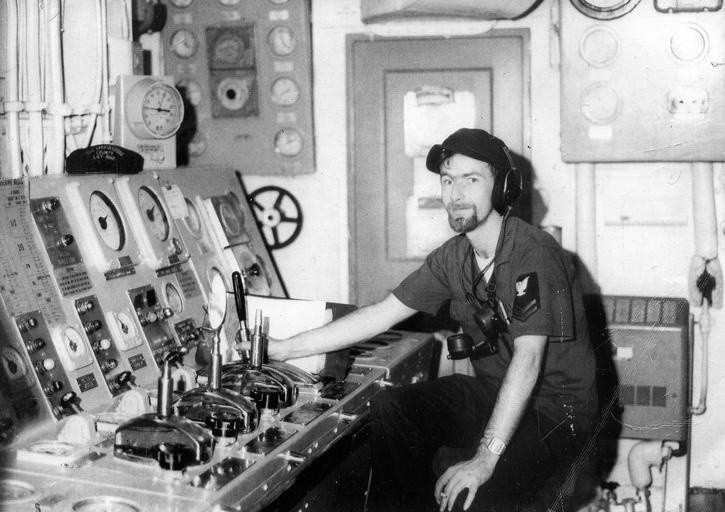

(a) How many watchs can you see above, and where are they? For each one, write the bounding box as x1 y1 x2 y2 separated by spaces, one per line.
479 436 507 455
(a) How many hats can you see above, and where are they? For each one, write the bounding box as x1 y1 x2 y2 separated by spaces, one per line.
425 129 512 172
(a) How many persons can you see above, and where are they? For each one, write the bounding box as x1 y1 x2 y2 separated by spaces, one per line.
233 128 600 512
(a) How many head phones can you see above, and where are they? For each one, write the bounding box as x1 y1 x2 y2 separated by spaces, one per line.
491 136 524 216
447 302 503 360
